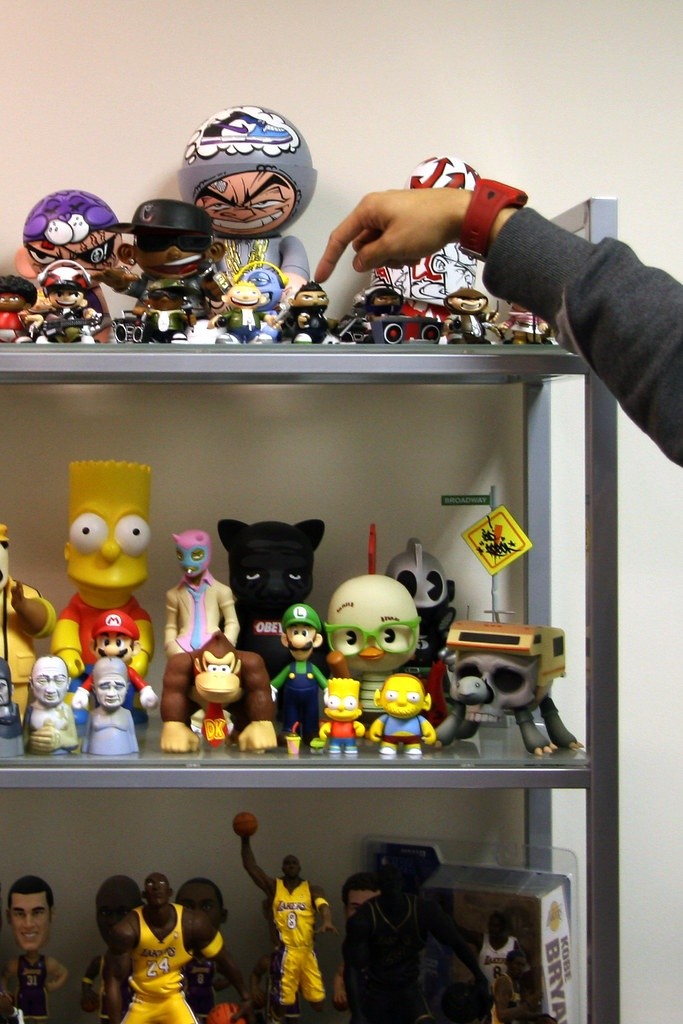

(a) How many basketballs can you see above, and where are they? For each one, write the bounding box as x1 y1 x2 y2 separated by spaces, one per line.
207 1003 243 1024
233 811 258 836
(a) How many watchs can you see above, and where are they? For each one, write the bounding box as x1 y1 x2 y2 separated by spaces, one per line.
460 179 528 262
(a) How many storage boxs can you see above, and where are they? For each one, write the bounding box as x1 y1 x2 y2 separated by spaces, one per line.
419 864 578 1024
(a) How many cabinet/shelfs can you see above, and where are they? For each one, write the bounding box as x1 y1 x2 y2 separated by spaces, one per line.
0 196 622 1024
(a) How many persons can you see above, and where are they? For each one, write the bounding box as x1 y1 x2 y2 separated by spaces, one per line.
27 654 79 754
334 855 489 1024
289 281 329 344
370 673 436 755
365 287 403 344
82 656 140 754
91 199 224 343
499 301 548 345
73 610 158 710
0 523 56 723
0 190 132 344
208 281 283 344
241 836 339 1024
443 289 498 344
456 911 556 1024
314 188 683 467
81 873 253 1024
0 876 69 1024
270 603 327 746
165 529 240 658
0 656 24 758
319 677 366 754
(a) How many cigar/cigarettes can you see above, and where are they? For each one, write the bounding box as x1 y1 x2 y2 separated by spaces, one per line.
187 569 191 574
